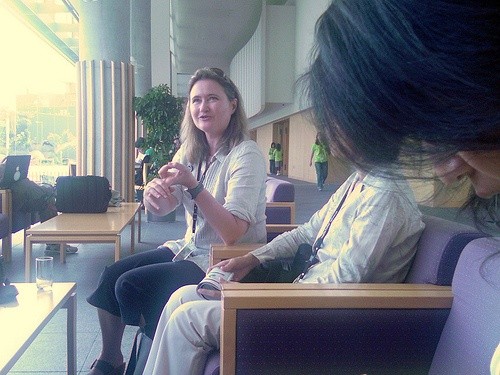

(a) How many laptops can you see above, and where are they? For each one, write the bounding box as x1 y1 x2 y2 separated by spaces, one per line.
0 155 31 188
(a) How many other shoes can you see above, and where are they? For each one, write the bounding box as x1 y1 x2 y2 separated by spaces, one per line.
317 186 323 191
45 245 78 253
276 171 280 175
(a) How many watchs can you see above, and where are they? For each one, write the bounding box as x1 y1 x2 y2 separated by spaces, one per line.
183 174 205 200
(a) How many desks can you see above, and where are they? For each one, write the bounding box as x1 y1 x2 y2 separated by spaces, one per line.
24 201 143 282
0 282 79 375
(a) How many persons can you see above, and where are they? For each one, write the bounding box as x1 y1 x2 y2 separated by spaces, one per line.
135 137 149 202
268 142 276 175
87 66 267 375
142 124 425 375
0 156 78 253
273 143 283 176
310 132 329 191
291 0 500 244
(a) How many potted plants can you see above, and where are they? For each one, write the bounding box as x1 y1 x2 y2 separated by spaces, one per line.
133 83 185 222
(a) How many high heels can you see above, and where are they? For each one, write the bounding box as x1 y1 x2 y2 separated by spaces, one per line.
85 359 126 375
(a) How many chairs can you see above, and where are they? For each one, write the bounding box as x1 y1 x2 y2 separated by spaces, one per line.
202 177 500 375
0 182 57 264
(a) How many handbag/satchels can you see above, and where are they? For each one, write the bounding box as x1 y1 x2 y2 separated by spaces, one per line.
56 175 111 213
239 242 312 283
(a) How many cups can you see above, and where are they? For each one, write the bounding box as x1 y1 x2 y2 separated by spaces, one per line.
36 257 54 289
